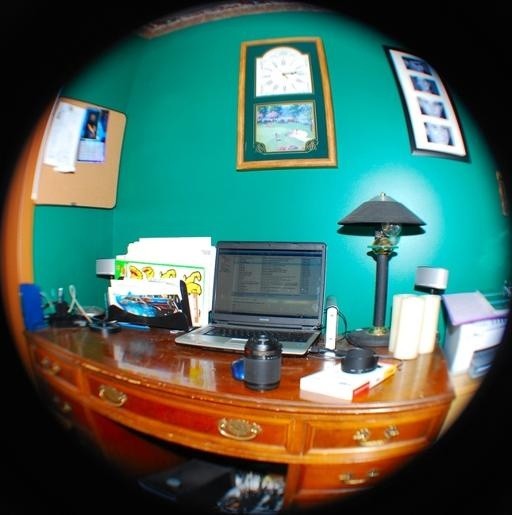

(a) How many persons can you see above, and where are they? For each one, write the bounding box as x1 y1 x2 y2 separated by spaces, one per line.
401 55 453 146
85 113 98 138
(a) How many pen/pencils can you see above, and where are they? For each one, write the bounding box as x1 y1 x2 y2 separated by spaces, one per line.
49 288 64 303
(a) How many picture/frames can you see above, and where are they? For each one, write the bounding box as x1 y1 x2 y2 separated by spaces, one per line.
381 43 474 164
234 36 343 172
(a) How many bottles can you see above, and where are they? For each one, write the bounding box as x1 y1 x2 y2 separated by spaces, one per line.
243 331 283 389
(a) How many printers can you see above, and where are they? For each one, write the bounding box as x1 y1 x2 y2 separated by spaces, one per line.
442 287 512 378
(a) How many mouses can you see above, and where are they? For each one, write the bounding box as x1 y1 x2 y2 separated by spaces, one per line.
342 348 379 373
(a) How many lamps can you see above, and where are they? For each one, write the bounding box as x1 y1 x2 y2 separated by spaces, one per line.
337 191 426 349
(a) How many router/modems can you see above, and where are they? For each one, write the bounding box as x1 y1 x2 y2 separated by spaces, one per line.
326 295 339 350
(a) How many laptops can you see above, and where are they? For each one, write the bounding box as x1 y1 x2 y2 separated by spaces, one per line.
175 241 326 357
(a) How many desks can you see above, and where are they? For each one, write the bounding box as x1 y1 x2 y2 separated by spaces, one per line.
23 314 458 513
